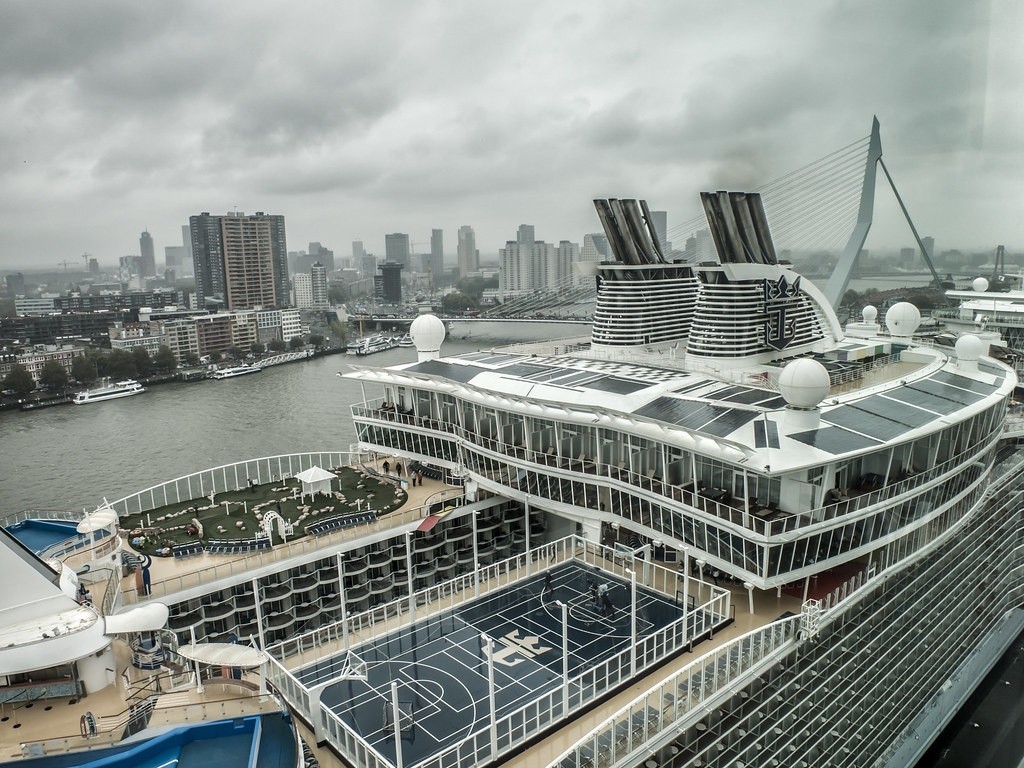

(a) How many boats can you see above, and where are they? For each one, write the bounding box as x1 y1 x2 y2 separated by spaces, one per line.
399 333 415 347
214 363 261 379
346 332 401 355
73 379 148 405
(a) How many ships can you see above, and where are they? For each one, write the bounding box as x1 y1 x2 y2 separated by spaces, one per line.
0 191 1024 768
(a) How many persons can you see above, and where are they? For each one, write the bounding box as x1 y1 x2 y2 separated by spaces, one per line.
586 578 616 616
79 583 86 595
600 537 618 560
383 460 390 474
276 502 281 515
545 570 555 592
395 462 402 477
85 590 92 606
193 503 200 517
247 479 255 493
676 559 684 582
417 469 422 486
411 470 416 487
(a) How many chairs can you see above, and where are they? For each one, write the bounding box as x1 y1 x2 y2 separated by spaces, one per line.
373 401 791 529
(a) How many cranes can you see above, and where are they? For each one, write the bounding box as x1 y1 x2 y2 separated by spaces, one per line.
81 252 92 278
57 260 80 269
